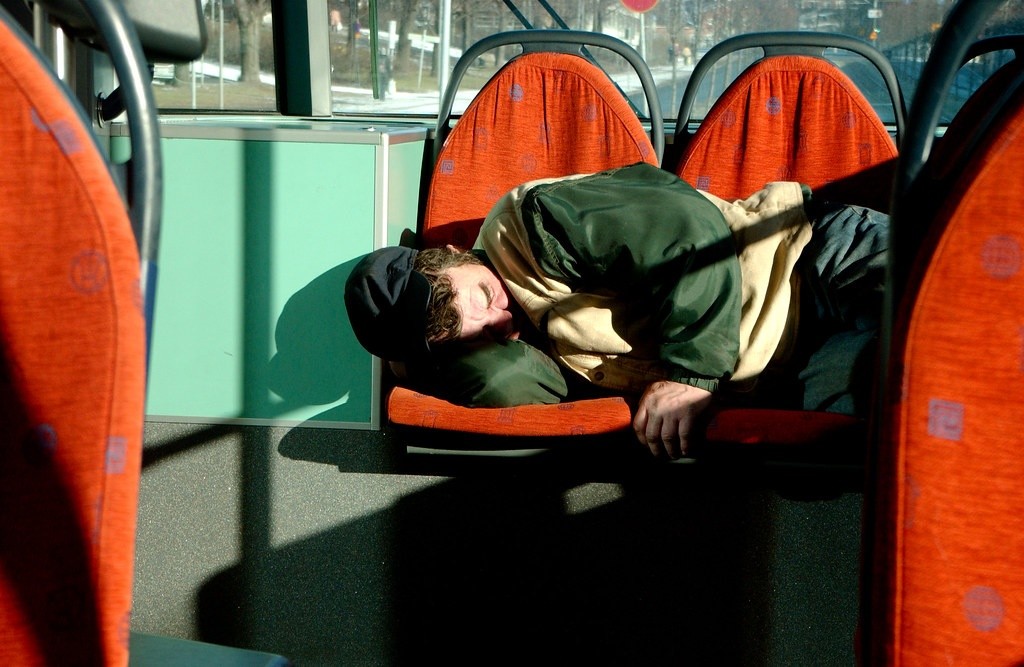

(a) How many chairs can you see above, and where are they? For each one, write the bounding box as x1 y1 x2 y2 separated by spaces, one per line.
382 26 1024 667
0 0 166 667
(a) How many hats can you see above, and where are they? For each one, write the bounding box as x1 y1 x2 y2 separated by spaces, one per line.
344 246 431 362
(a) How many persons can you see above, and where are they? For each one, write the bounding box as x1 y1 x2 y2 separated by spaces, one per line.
669 44 691 65
344 163 890 459
